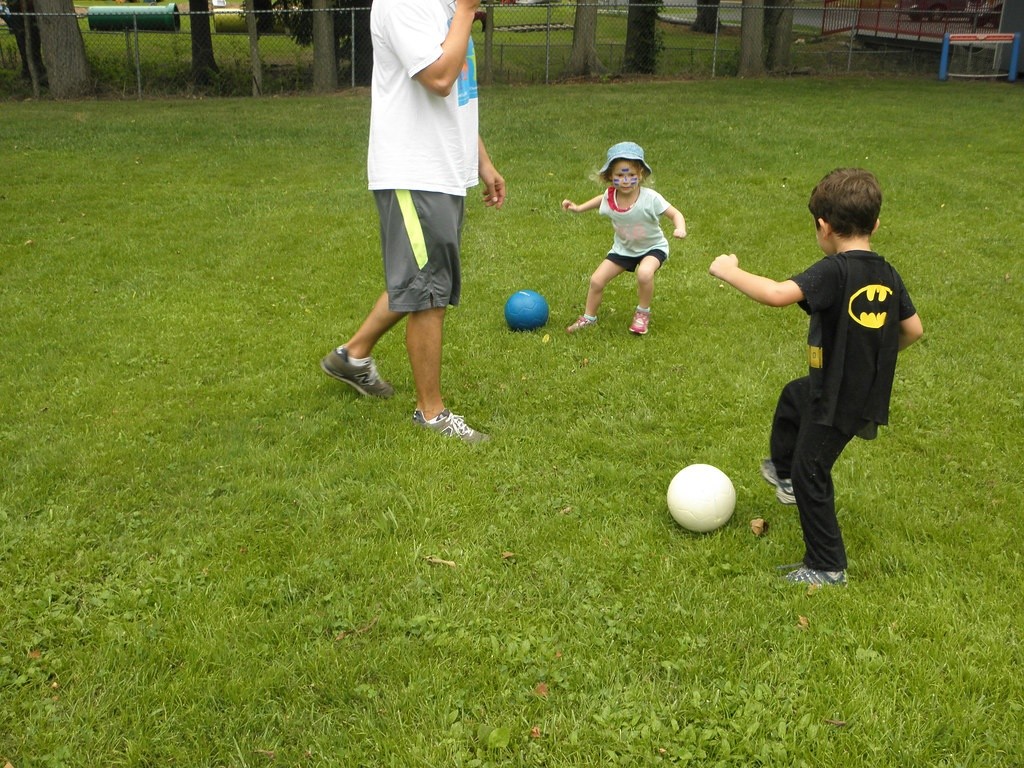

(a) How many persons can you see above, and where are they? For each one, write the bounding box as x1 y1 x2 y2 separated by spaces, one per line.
319 0 507 444
709 167 924 586
562 141 688 334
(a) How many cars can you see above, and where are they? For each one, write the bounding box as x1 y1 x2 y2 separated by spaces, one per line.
893 1 1002 32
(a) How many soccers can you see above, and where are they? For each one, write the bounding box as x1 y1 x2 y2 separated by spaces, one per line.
667 464 737 534
504 289 550 331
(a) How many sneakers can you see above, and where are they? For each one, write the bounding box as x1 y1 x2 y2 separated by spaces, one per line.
566 315 598 333
785 565 848 588
320 344 394 400
412 408 492 447
629 308 650 334
760 458 797 504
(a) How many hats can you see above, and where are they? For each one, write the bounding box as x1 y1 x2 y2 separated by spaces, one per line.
598 142 652 177
